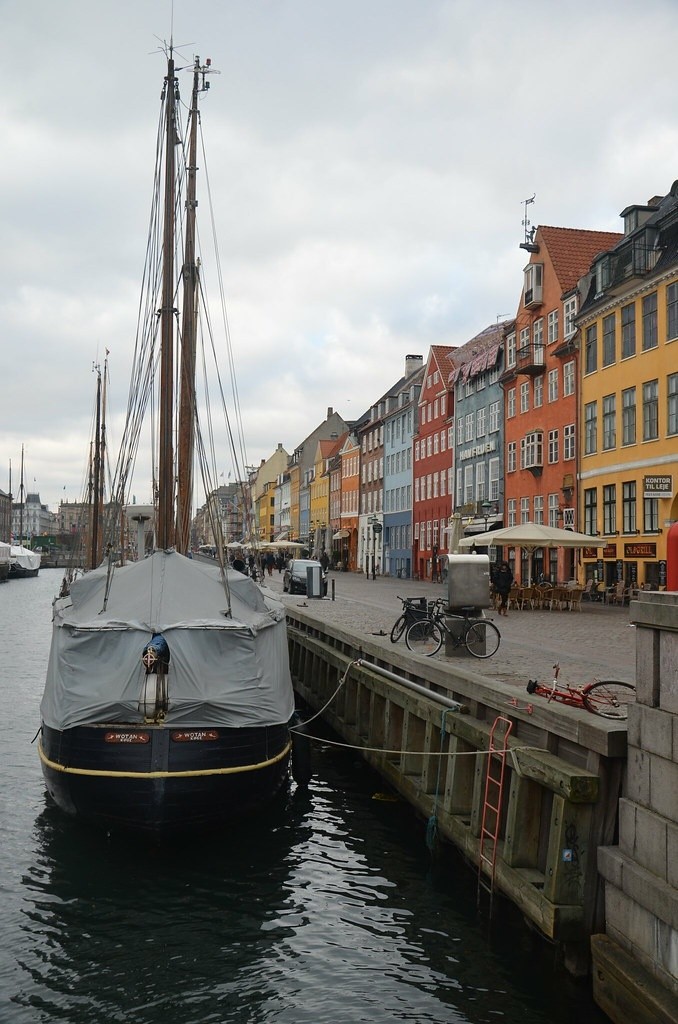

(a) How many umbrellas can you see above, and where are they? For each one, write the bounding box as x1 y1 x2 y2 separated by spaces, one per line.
262 540 306 561
458 522 608 588
444 513 464 570
226 540 286 550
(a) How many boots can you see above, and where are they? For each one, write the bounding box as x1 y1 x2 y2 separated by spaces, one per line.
497 605 502 615
503 607 508 616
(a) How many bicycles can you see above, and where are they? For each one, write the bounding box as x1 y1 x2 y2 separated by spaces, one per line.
526 660 637 720
390 594 502 658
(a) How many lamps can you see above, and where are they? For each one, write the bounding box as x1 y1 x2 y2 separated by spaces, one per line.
493 502 499 514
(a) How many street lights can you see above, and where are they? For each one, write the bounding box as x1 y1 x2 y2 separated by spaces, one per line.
371 514 379 580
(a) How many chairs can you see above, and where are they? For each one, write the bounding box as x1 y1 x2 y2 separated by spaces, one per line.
491 586 584 612
584 579 651 607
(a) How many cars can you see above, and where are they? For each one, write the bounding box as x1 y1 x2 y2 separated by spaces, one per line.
282 558 327 597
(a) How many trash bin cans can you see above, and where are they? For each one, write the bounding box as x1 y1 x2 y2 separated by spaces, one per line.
407 597 427 639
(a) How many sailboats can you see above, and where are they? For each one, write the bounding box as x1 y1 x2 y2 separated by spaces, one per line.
0 443 43 581
30 33 308 835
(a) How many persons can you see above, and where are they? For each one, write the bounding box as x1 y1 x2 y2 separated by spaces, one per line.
260 553 275 576
320 552 330 571
249 554 255 572
493 561 513 617
277 555 284 573
233 552 245 572
